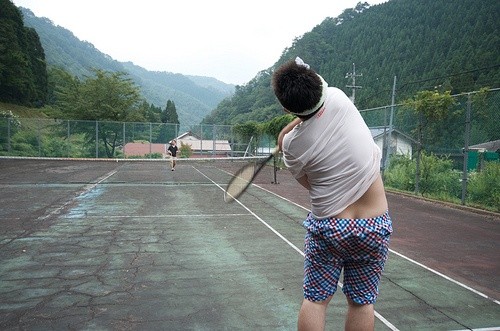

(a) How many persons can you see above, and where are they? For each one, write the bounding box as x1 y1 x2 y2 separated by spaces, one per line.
167 139 178 171
270 57 393 330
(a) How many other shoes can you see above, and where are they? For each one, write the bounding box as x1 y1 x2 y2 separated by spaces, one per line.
171 168 175 171
